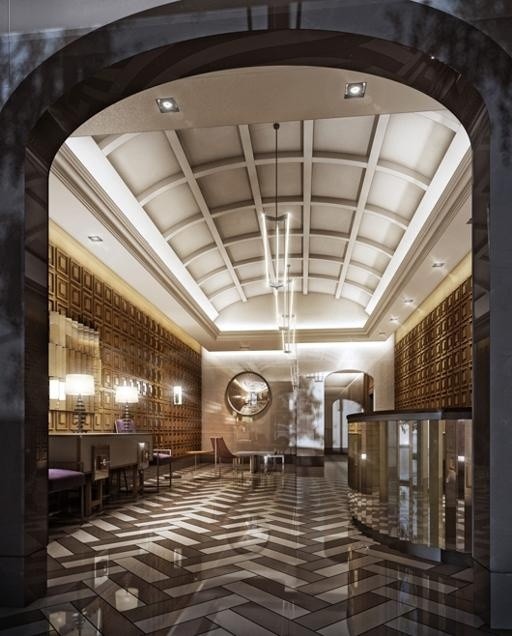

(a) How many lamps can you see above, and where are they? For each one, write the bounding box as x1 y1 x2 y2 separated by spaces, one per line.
262 214 300 393
50 373 138 432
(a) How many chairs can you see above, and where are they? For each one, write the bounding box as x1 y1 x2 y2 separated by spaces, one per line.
46 438 176 520
208 433 295 478
